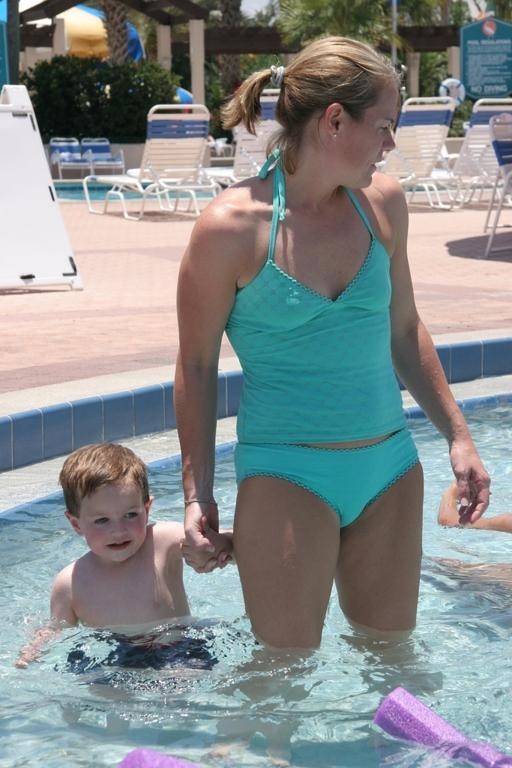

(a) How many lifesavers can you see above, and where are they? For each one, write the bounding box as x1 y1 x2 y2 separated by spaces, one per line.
439 78 465 105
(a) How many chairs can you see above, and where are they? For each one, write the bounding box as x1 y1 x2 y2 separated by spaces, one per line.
81 90 282 226
49 132 127 178
378 90 512 257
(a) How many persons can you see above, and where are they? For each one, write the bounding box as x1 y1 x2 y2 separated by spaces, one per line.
12 443 237 703
428 480 511 588
171 34 492 656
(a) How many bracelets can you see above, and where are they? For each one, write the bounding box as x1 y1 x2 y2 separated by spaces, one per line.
183 497 220 508
